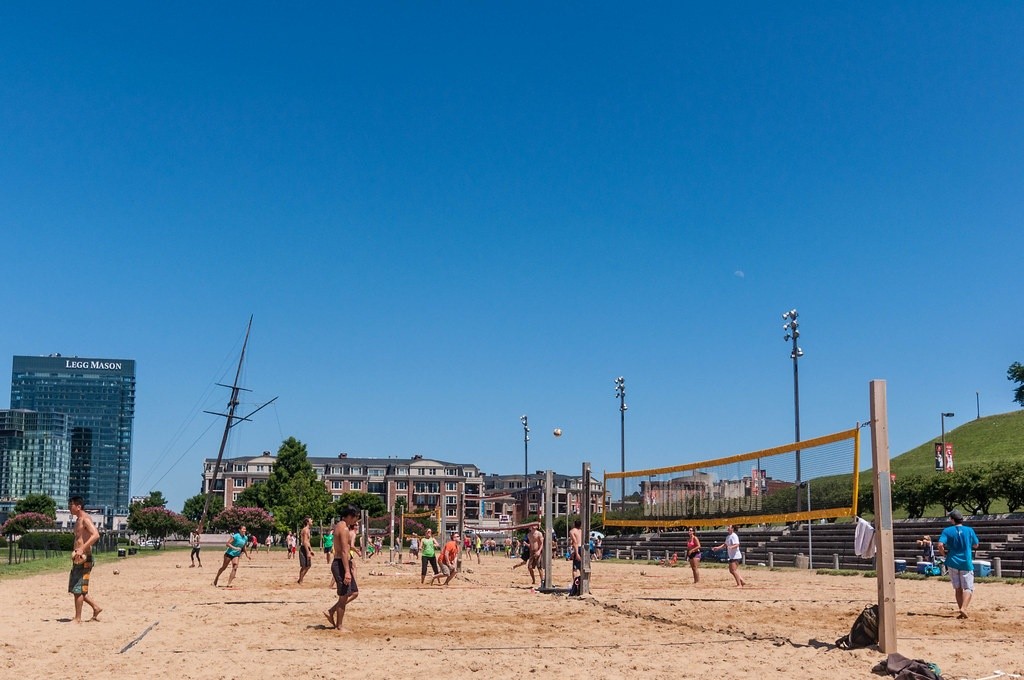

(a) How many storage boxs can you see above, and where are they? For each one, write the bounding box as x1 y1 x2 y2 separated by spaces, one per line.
917 562 932 573
972 559 991 577
895 559 906 571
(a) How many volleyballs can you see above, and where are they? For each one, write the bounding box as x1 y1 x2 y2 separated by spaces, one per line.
113 569 120 575
554 428 562 436
71 549 88 565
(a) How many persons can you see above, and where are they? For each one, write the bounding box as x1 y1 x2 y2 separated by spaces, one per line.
569 520 582 583
552 539 557 561
68 496 103 623
431 533 460 586
523 525 544 585
687 528 701 584
673 551 678 561
360 533 421 559
213 525 251 587
323 504 359 633
589 533 602 561
297 517 314 585
917 535 934 563
250 531 296 559
323 529 334 564
189 531 202 568
712 525 746 587
465 534 530 571
419 528 441 584
938 510 979 618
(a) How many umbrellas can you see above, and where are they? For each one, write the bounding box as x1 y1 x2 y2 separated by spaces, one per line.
589 531 604 540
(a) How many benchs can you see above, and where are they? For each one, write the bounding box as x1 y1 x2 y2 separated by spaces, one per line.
557 519 1024 577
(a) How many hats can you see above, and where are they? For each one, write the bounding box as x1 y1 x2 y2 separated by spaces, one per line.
948 509 963 520
338 504 359 517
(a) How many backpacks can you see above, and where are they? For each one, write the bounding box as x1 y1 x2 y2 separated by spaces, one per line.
569 576 580 596
836 603 879 650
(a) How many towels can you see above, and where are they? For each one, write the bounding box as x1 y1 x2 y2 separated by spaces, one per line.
854 518 876 559
